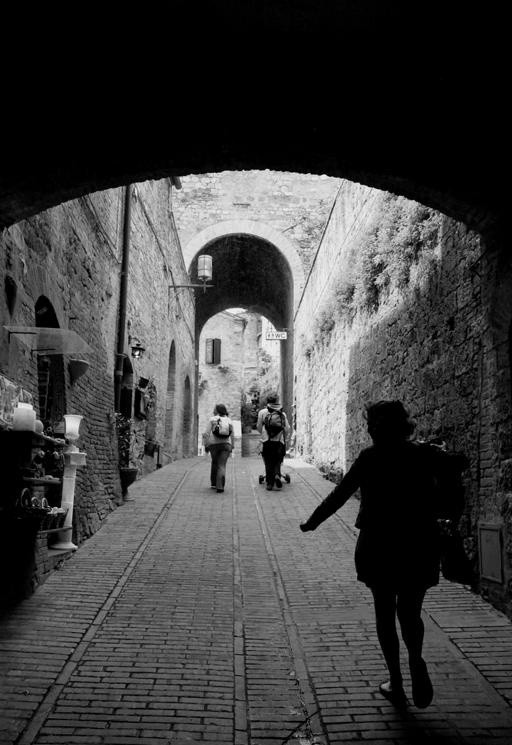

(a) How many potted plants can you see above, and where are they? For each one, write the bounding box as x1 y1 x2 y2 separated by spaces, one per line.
114 410 140 488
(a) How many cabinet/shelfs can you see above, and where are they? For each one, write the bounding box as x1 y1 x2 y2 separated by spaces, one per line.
1 429 78 541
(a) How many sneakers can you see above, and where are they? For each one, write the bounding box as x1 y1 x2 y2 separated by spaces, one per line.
266 484 273 490
275 473 283 488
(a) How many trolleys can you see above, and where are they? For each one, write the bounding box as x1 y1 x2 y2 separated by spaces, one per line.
259 427 291 484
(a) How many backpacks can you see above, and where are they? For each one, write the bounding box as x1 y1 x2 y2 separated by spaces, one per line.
212 415 232 438
262 408 286 442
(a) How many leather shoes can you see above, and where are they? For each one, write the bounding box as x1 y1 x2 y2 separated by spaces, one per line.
379 679 409 704
410 659 434 709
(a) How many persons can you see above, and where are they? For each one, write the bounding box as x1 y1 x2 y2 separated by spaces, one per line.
257 395 290 490
203 404 234 493
300 400 455 709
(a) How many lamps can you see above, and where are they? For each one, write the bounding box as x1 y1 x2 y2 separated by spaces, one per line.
168 254 215 293
127 335 146 360
62 413 84 452
